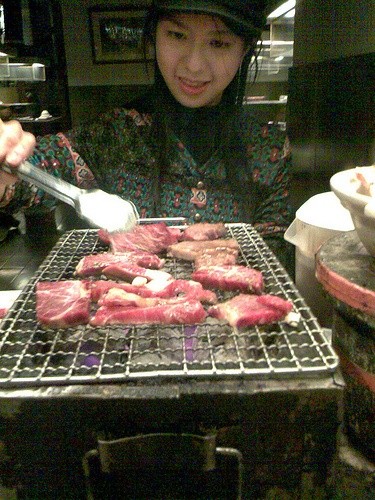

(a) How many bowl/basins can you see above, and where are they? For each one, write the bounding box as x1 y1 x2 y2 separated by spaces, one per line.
329 165 375 255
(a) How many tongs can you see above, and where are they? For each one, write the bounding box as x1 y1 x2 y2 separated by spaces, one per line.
0 157 140 232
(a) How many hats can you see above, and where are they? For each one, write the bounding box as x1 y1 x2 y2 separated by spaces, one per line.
154 1 266 34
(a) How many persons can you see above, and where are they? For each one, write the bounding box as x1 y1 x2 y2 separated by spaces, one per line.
1 0 295 276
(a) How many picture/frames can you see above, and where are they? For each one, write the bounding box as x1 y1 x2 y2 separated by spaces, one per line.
89 6 156 64
